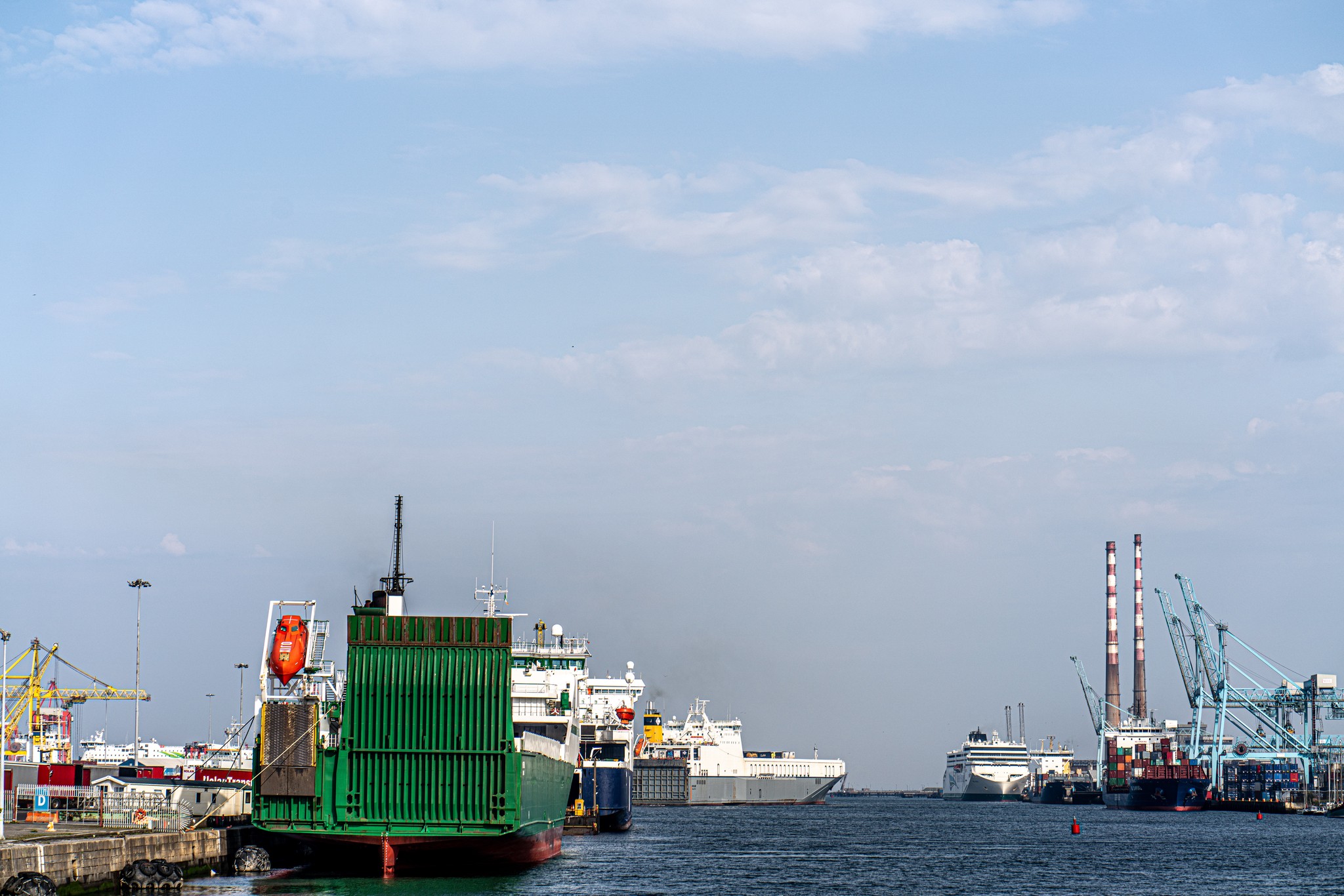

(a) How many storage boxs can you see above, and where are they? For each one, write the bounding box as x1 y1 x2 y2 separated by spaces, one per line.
1107 739 1344 802
1034 774 1089 796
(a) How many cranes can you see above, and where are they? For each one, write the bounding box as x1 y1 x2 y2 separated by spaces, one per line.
1068 573 1344 769
1 637 151 764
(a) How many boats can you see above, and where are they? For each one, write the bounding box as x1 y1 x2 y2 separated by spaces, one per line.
1102 740 1211 812
271 614 308 686
1021 735 1075 802
942 726 1032 803
1039 764 1104 805
0 493 649 879
631 695 846 806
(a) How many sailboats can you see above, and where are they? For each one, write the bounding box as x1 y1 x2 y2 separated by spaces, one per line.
1296 739 1344 818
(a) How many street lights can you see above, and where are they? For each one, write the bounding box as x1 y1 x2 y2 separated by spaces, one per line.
205 693 215 744
127 578 152 766
0 627 12 840
234 663 249 750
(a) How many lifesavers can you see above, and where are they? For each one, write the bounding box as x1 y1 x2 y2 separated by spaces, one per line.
552 708 558 715
118 858 183 888
232 845 271 871
0 871 55 896
136 808 146 818
